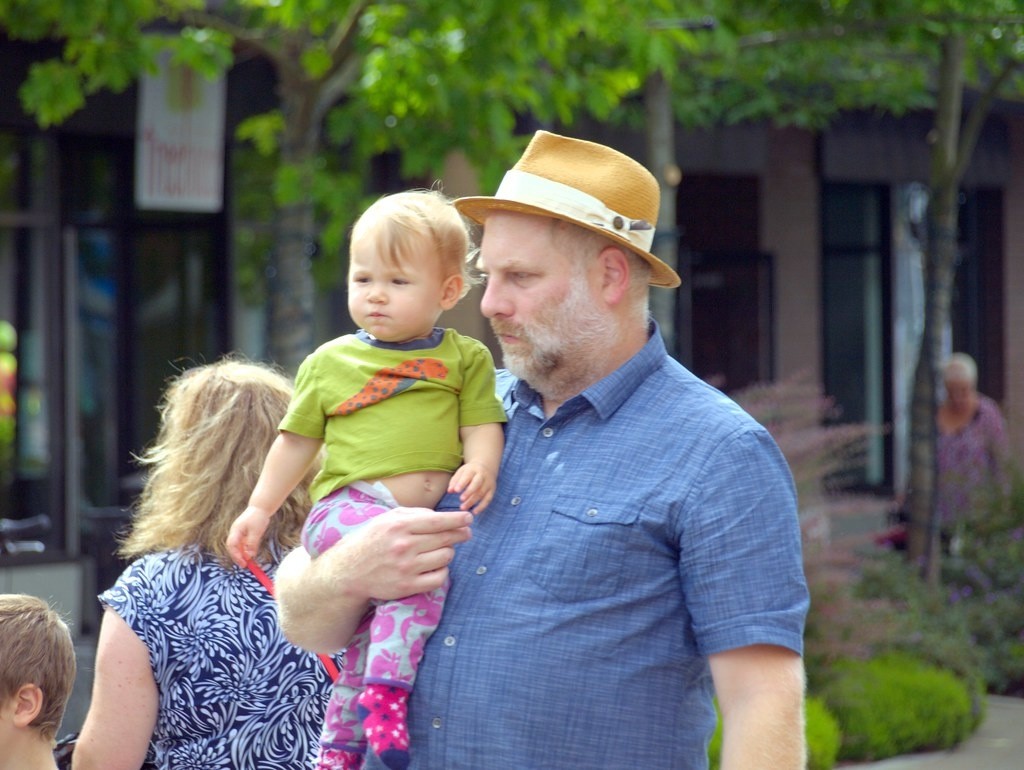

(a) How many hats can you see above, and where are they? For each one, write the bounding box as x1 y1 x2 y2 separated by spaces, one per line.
451 130 681 288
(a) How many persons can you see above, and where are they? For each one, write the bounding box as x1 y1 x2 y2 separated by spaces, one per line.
0 593 76 770
902 354 1008 561
71 355 351 770
225 188 511 770
275 130 811 770
